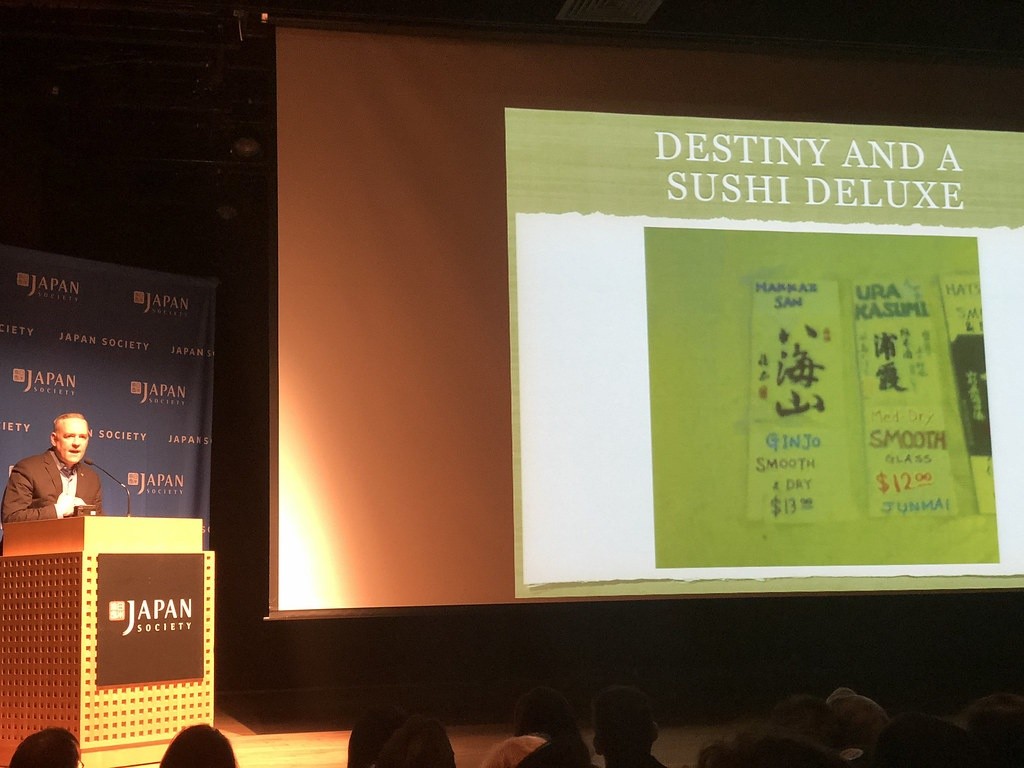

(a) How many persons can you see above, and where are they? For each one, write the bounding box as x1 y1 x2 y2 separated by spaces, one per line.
159 724 239 768
770 686 894 750
593 687 668 768
347 668 456 768
1 413 103 523
698 723 851 768
8 727 85 768
875 693 1024 768
481 687 592 768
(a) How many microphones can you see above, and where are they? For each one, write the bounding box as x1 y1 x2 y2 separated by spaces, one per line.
84 458 130 517
